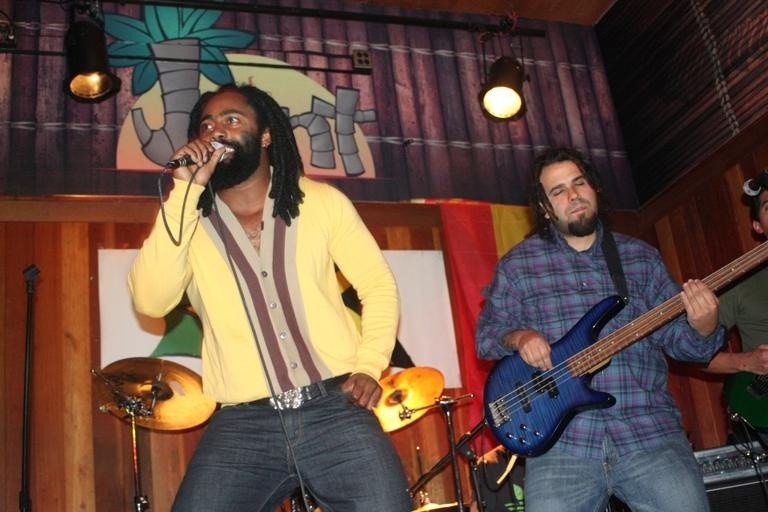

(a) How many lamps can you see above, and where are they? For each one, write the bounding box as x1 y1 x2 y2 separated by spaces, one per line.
478 27 532 123
62 2 122 105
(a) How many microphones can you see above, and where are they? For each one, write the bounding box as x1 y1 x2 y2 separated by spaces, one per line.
741 169 767 205
166 141 225 170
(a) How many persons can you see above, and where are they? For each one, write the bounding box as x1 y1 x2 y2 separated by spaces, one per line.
671 172 767 448
127 82 415 512
473 145 727 511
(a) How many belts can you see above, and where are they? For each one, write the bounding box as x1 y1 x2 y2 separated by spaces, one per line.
235 371 351 411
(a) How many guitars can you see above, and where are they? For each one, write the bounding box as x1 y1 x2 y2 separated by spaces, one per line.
483 242 768 457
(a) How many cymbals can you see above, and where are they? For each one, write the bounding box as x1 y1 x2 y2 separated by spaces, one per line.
372 368 445 434
93 356 217 431
